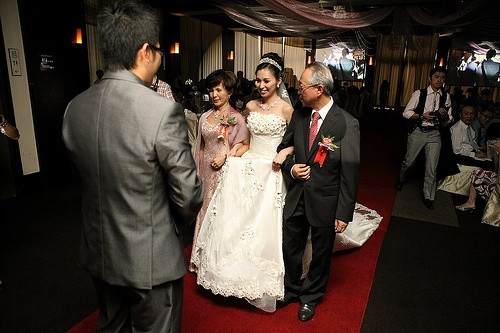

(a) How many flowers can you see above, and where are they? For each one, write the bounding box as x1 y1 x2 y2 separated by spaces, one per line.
220 113 237 128
318 132 340 152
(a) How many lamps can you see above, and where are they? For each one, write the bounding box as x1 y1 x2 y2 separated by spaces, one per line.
369 57 375 65
230 51 233 60
175 43 179 54
308 55 311 64
439 58 444 67
76 28 82 44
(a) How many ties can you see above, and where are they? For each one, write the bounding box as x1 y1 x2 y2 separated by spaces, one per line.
308 113 321 154
467 127 479 151
432 92 437 111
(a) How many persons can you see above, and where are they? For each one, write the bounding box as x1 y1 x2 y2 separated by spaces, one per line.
237 71 248 82
189 52 384 313
61 0 205 333
150 75 176 102
396 66 454 210
94 69 103 84
449 101 500 213
189 70 249 272
0 112 20 140
276 62 360 322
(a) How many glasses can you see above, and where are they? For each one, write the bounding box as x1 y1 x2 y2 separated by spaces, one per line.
149 44 164 57
297 84 316 92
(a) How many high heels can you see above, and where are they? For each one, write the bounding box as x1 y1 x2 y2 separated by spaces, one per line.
456 205 476 213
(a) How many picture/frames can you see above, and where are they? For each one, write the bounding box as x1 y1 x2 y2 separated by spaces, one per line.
8 48 22 76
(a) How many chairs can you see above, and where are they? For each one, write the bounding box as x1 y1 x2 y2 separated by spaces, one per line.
439 106 492 198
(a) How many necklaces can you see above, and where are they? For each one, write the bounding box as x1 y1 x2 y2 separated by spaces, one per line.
212 109 226 122
259 97 280 110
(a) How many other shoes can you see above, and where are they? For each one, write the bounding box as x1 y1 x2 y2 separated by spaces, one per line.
423 199 434 208
396 181 404 191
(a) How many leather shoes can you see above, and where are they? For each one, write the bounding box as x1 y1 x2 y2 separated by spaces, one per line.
276 297 293 307
298 304 315 320
